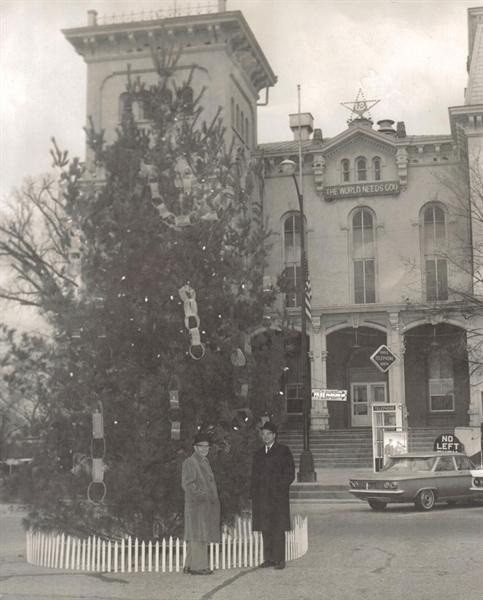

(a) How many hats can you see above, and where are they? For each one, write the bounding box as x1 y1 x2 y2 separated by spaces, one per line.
193 434 209 446
260 423 275 432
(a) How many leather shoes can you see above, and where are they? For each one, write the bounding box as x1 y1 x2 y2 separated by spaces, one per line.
261 561 285 569
184 567 212 575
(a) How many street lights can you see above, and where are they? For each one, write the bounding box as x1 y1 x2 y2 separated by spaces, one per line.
276 158 320 485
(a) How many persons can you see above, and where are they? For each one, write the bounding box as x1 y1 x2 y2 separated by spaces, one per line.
178 434 220 577
248 422 292 570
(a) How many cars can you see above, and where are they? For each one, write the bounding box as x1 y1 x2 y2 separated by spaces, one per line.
468 463 483 492
344 450 480 514
